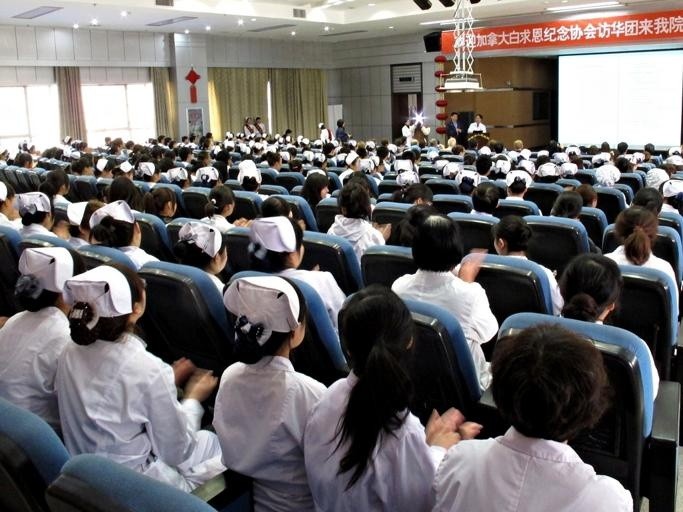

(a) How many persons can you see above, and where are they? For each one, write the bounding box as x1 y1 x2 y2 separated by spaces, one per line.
433 322 634 511
317 122 333 142
401 119 415 145
55 263 228 492
446 112 465 145
467 114 486 134
242 116 256 137
210 275 328 511
0 246 85 443
336 120 350 142
253 116 265 137
303 284 483 512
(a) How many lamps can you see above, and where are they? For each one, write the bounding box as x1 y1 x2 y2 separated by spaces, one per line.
413 0 481 11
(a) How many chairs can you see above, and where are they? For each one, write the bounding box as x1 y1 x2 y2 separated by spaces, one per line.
0 142 682 511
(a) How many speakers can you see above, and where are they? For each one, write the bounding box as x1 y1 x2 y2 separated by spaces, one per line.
423 32 442 52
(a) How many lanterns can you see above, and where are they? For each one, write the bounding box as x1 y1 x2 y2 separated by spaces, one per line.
433 71 446 82
434 99 448 109
433 55 446 68
435 86 446 95
435 112 448 124
435 127 447 137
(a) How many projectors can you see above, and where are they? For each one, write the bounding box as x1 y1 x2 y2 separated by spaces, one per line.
445 78 480 89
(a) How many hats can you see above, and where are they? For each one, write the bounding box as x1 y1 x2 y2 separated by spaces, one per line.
16 245 73 298
0 181 7 202
11 189 53 216
62 116 683 199
0 138 45 157
62 263 136 320
86 199 135 231
175 221 226 258
248 215 298 253
221 272 302 333
